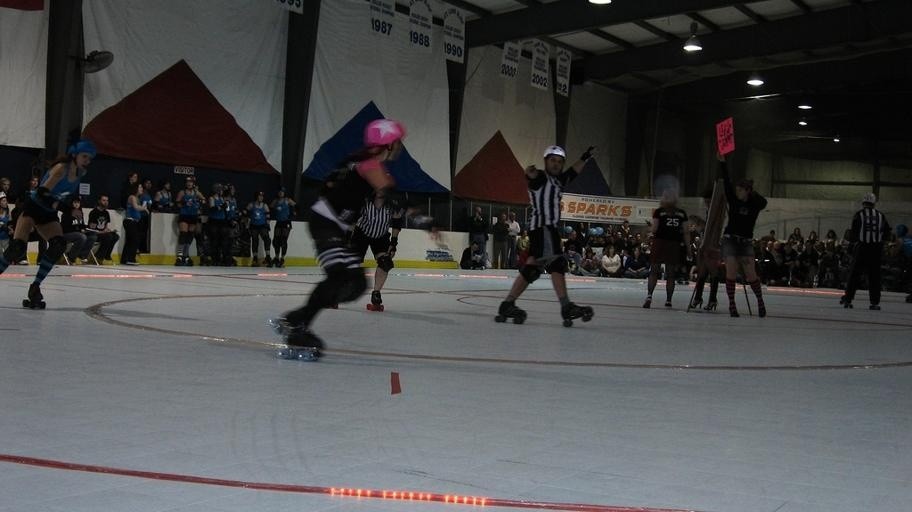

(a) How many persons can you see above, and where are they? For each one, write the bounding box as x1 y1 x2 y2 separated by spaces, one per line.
499 145 592 317
641 188 691 307
715 149 768 317
1 168 299 268
280 117 408 354
0 138 97 302
837 193 890 307
393 193 912 300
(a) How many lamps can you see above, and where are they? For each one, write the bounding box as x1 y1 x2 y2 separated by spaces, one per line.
747 67 765 86
683 21 703 52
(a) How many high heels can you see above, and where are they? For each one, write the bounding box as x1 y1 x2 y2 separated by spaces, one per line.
704 302 717 310
690 299 703 309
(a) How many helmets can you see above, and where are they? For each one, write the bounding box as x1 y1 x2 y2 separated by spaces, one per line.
186 175 197 182
367 118 405 144
66 141 95 157
212 182 233 192
565 226 572 233
543 146 566 160
861 192 875 203
588 226 602 236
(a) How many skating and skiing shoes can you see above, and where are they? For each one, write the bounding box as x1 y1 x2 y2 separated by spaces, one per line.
870 298 880 309
560 303 593 326
366 290 384 312
199 255 238 266
495 300 526 324
252 255 284 268
840 295 853 309
269 313 322 362
176 255 193 266
23 283 46 309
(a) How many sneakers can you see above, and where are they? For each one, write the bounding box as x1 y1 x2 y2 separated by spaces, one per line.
643 299 651 309
759 303 766 318
729 306 739 317
